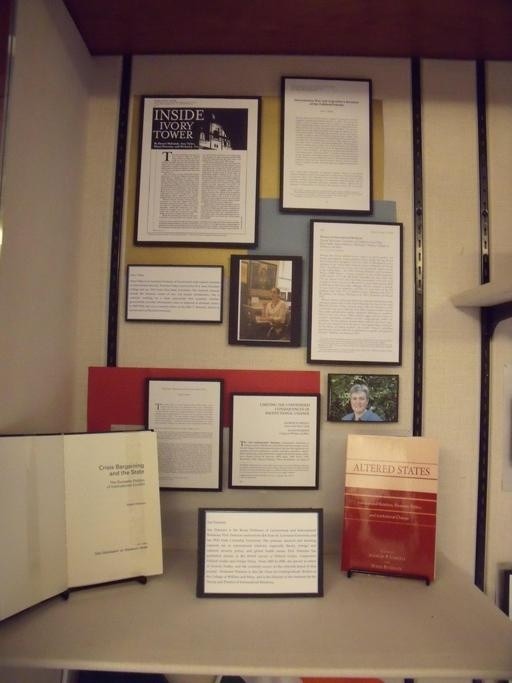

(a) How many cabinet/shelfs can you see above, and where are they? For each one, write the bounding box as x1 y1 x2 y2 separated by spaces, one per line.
0 0 512 683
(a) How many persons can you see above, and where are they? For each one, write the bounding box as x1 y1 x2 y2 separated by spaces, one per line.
257 287 289 335
240 282 257 339
342 383 383 421
